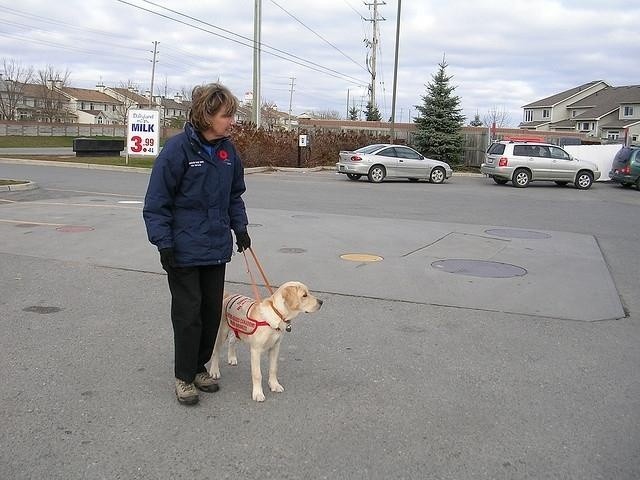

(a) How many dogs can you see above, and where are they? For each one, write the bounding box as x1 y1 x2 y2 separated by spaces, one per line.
208 281 323 403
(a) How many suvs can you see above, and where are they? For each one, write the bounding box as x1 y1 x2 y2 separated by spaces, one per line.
480 136 601 190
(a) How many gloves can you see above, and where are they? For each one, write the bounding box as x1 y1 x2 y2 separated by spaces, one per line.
236 232 250 252
159 248 176 272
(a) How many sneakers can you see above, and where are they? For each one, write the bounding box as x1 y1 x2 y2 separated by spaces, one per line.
174 377 199 405
194 372 219 392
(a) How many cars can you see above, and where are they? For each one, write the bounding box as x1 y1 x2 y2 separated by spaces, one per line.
335 143 455 184
609 143 640 191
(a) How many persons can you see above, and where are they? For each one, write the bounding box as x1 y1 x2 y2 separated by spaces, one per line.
142 82 252 406
